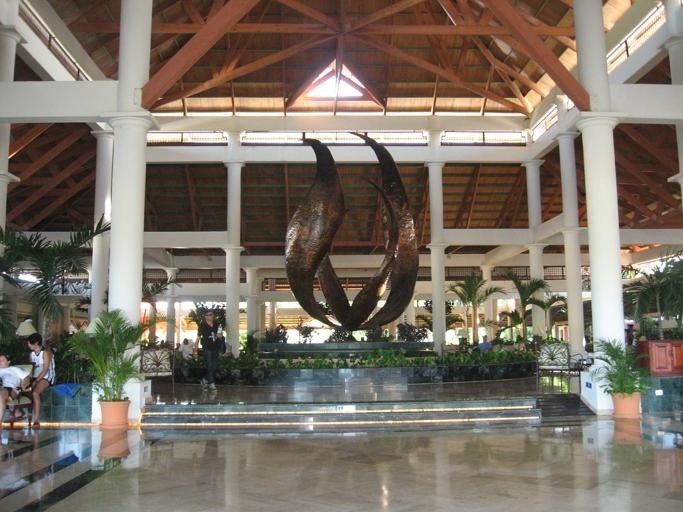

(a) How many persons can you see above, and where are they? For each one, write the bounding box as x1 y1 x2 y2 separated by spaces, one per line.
11 333 57 430
0 353 29 426
632 330 647 344
193 309 223 391
179 338 193 360
477 335 491 351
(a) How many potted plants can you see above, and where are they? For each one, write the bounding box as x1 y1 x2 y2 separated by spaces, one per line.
591 339 656 419
61 307 177 458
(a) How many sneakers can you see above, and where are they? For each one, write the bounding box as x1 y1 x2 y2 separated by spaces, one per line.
33 422 40 429
201 379 218 392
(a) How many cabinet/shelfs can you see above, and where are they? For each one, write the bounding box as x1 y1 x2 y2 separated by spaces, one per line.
634 340 683 376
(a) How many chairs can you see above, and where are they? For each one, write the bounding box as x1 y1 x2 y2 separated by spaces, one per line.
2 361 36 429
